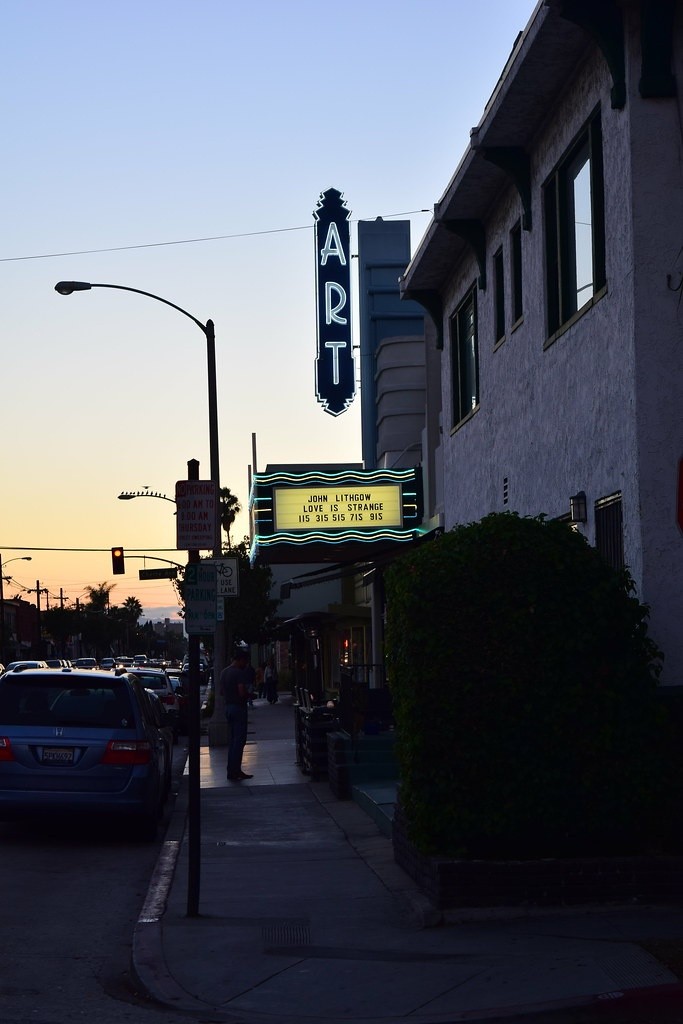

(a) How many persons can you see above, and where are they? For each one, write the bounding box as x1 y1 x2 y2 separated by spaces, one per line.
263 659 279 705
255 662 267 699
218 647 254 785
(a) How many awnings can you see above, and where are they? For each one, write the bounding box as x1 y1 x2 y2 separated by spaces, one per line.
280 562 378 601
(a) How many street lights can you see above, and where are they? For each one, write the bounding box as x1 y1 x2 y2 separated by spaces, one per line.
53 279 232 918
113 458 209 637
0 553 32 668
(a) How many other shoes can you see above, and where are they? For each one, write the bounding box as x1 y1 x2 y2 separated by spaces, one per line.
239 772 254 779
228 772 242 782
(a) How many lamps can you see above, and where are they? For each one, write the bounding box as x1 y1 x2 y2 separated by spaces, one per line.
570 490 589 523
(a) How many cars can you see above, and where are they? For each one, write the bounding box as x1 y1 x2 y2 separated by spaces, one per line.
165 663 207 709
1 646 180 676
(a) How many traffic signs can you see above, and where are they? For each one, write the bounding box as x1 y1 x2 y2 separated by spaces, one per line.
137 566 179 581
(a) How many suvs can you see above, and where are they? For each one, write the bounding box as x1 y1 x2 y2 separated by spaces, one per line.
0 663 174 848
111 664 183 747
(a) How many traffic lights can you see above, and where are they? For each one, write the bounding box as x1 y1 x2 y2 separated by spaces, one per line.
109 547 126 576
